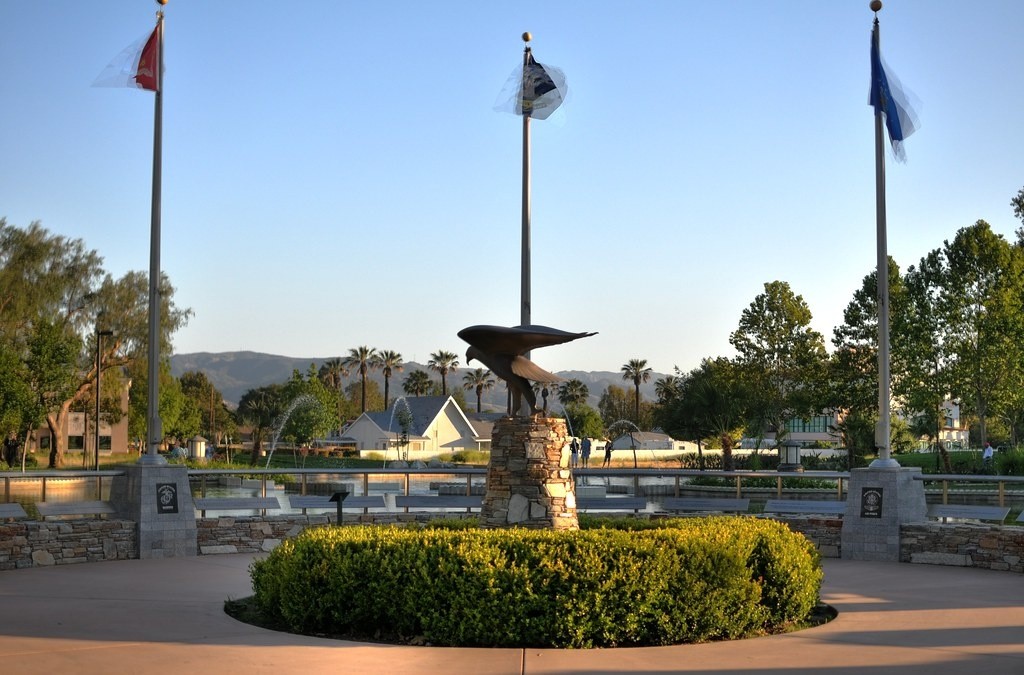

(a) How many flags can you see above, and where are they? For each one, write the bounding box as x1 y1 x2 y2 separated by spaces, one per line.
87 20 162 93
865 25 919 160
498 47 569 121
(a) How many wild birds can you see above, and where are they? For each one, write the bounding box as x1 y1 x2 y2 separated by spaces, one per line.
457 323 600 424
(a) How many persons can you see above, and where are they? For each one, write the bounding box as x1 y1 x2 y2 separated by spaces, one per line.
600 436 613 468
570 438 579 468
980 440 994 460
580 436 591 468
